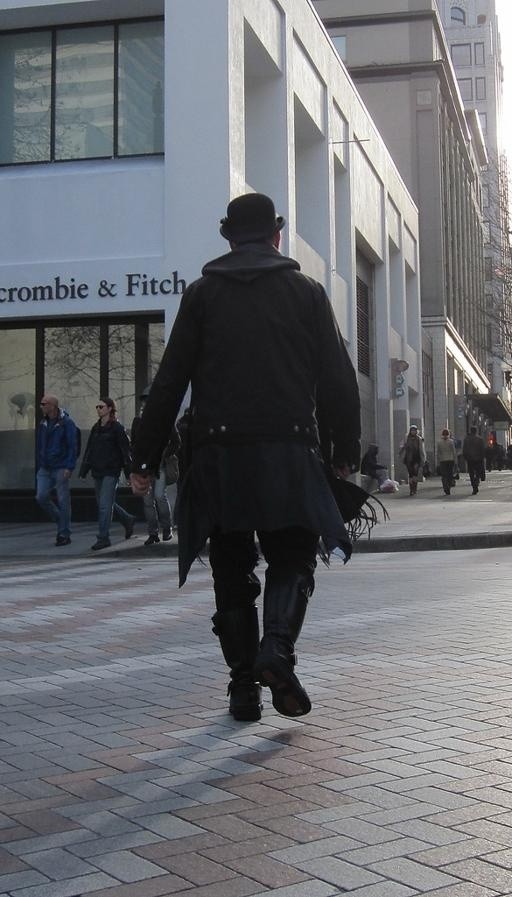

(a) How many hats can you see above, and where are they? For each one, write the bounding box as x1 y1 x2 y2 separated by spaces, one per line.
218 191 285 246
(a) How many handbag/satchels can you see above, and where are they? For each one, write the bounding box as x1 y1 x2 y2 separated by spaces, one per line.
163 454 179 485
480 460 485 481
422 461 432 478
452 465 459 486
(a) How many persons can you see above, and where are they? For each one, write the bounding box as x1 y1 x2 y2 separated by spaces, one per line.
399 425 426 495
463 427 484 494
361 445 387 482
435 429 457 496
35 394 80 546
484 442 511 471
130 393 183 546
78 397 134 548
128 194 360 720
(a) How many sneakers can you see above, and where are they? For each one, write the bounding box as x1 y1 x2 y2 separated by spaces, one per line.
471 488 479 495
443 488 451 496
54 534 71 546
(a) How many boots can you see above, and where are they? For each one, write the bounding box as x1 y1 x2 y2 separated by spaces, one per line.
121 514 136 540
410 481 417 496
258 565 315 717
211 606 265 721
89 534 112 551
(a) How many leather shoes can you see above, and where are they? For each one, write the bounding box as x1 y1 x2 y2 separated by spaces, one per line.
143 535 160 546
163 527 172 541
